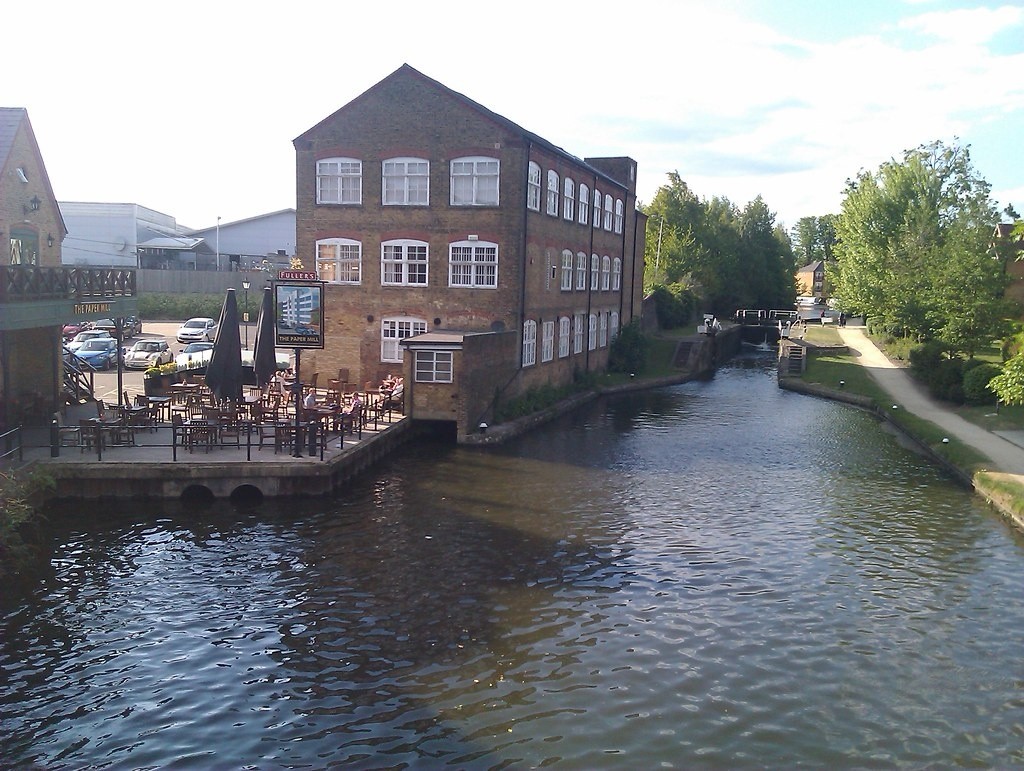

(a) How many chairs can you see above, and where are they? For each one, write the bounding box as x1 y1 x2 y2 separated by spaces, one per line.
54 369 404 453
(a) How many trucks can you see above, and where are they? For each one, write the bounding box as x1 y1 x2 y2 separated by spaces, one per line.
295 325 309 333
278 320 289 328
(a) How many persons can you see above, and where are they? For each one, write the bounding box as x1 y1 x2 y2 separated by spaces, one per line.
305 388 317 407
378 377 404 407
271 371 288 404
350 392 362 417
821 309 826 326
839 312 846 327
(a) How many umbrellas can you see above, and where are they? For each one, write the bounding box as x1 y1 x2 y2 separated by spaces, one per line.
252 286 277 395
204 288 243 401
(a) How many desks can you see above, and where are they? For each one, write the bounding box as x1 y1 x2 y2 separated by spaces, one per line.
89 417 123 447
120 405 148 431
183 418 219 450
215 407 246 436
309 405 341 432
280 376 295 401
365 389 393 423
278 421 309 447
172 383 200 405
133 397 172 423
227 397 263 420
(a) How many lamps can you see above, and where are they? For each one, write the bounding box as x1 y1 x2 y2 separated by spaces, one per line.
942 437 949 444
479 421 487 435
48 233 54 247
114 237 125 251
891 404 898 410
23 194 41 215
840 380 844 386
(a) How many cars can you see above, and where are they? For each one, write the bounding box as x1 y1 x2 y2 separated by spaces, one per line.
795 296 825 317
175 342 215 366
125 340 174 371
126 315 143 335
93 319 133 343
64 330 113 354
176 318 218 343
62 321 92 342
74 339 126 371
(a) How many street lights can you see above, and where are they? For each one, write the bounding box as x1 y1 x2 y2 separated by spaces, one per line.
242 277 252 350
217 216 222 272
652 214 664 268
